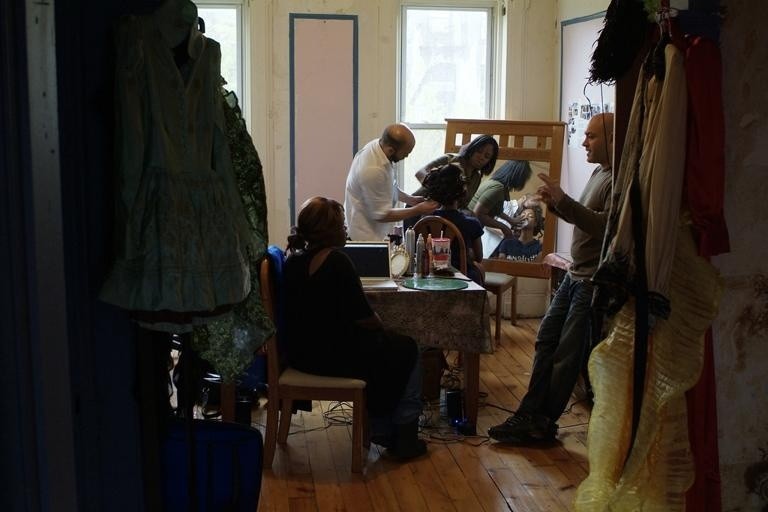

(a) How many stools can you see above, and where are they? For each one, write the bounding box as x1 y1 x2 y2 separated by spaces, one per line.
484 272 518 349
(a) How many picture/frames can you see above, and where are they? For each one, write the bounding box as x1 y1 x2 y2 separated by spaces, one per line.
552 6 617 264
289 12 357 240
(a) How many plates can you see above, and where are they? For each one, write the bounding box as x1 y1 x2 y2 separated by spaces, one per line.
400 277 469 291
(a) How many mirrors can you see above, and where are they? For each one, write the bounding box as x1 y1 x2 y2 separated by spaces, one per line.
445 120 566 277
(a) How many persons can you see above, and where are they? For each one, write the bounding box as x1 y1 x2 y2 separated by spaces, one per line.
402 132 498 240
284 195 428 462
496 204 544 261
467 159 532 238
485 112 615 444
341 122 438 243
480 193 539 259
422 162 486 289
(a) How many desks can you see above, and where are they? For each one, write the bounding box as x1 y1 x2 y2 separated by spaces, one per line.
357 262 486 437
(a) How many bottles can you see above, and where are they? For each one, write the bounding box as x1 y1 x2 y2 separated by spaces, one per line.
391 225 451 278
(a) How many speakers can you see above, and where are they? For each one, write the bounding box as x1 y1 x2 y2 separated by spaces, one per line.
235 401 251 426
445 389 462 426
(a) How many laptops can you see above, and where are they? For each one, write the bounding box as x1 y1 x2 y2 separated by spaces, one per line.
343 240 398 290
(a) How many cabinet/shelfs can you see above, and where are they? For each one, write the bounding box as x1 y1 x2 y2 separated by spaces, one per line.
547 253 575 305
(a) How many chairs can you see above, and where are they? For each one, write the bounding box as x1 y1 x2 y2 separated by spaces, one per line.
258 258 367 479
411 214 493 367
150 418 265 510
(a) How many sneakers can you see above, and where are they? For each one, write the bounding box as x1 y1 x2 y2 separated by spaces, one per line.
371 422 428 456
488 412 554 447
